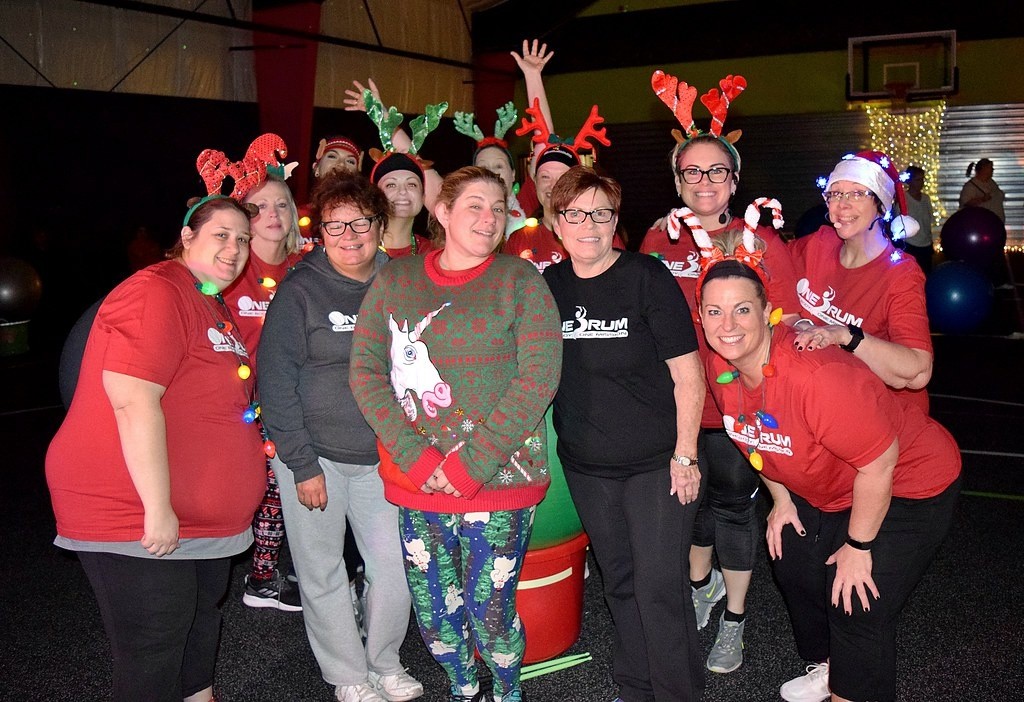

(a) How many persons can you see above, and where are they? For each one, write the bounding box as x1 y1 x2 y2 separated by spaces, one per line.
195 40 963 702
43 195 267 702
960 158 1007 227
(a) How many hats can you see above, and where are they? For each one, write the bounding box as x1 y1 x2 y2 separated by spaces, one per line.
825 150 920 239
535 146 578 176
372 152 424 196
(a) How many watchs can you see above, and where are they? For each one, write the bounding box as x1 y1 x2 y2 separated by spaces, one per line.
673 454 698 467
839 323 864 354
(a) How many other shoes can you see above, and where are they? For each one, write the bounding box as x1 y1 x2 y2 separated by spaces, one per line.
351 593 368 639
479 689 523 702
448 688 486 702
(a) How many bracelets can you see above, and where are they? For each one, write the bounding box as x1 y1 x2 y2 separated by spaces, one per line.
844 534 874 551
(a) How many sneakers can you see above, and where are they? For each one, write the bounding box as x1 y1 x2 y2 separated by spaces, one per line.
335 682 388 702
780 657 831 702
690 568 727 631
706 610 745 673
368 666 424 702
243 568 303 612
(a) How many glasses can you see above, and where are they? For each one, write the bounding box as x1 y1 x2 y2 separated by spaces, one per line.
679 168 735 184
823 190 875 202
319 213 382 236
557 209 616 224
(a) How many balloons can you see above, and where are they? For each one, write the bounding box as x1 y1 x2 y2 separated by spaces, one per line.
927 205 1007 331
